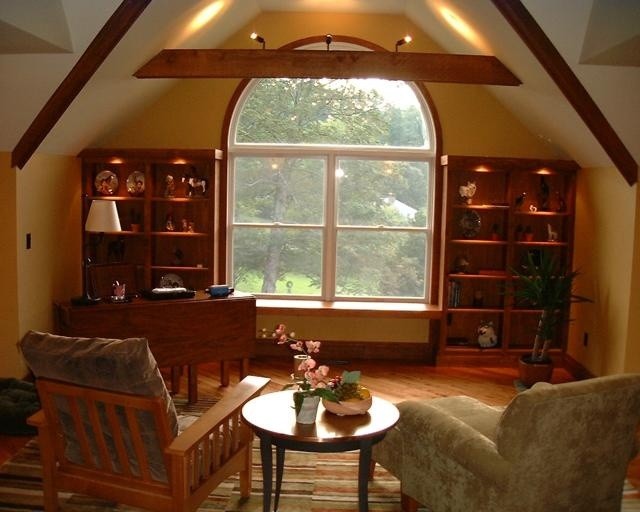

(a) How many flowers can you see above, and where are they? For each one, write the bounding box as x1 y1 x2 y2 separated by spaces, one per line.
259 324 341 416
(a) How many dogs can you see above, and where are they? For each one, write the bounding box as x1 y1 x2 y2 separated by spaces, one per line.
478 319 497 347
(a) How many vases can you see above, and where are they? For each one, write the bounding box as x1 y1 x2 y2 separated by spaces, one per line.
294 392 320 425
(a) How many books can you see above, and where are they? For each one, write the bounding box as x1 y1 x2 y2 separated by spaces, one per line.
447 279 463 309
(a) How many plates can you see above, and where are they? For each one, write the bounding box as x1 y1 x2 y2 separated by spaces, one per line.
154 270 192 292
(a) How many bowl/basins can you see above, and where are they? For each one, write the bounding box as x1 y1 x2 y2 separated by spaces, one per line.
322 394 372 416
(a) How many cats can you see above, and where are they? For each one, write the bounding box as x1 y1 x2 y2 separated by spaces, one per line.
459 181 477 205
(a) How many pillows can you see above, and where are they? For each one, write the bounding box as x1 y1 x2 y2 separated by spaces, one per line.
15 330 181 463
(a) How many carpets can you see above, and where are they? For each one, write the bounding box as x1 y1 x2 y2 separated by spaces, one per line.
0 433 640 512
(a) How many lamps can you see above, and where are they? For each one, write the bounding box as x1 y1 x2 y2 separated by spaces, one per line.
71 200 121 305
250 32 412 52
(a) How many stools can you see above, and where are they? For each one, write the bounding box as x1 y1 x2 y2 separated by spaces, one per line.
368 427 401 481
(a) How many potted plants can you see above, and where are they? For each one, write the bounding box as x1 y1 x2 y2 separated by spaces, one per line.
499 246 595 389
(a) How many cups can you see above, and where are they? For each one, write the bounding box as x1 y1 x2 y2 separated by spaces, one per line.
205 284 235 298
112 284 127 300
131 223 141 232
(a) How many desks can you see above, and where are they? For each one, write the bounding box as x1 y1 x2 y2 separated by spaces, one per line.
58 291 257 404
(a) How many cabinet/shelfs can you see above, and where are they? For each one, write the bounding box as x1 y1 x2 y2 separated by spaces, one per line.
81 147 224 296
433 154 577 366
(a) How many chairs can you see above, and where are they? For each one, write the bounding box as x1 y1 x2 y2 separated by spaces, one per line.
15 330 271 512
396 372 640 512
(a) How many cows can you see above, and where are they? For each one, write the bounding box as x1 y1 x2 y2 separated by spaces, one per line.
181 172 207 197
